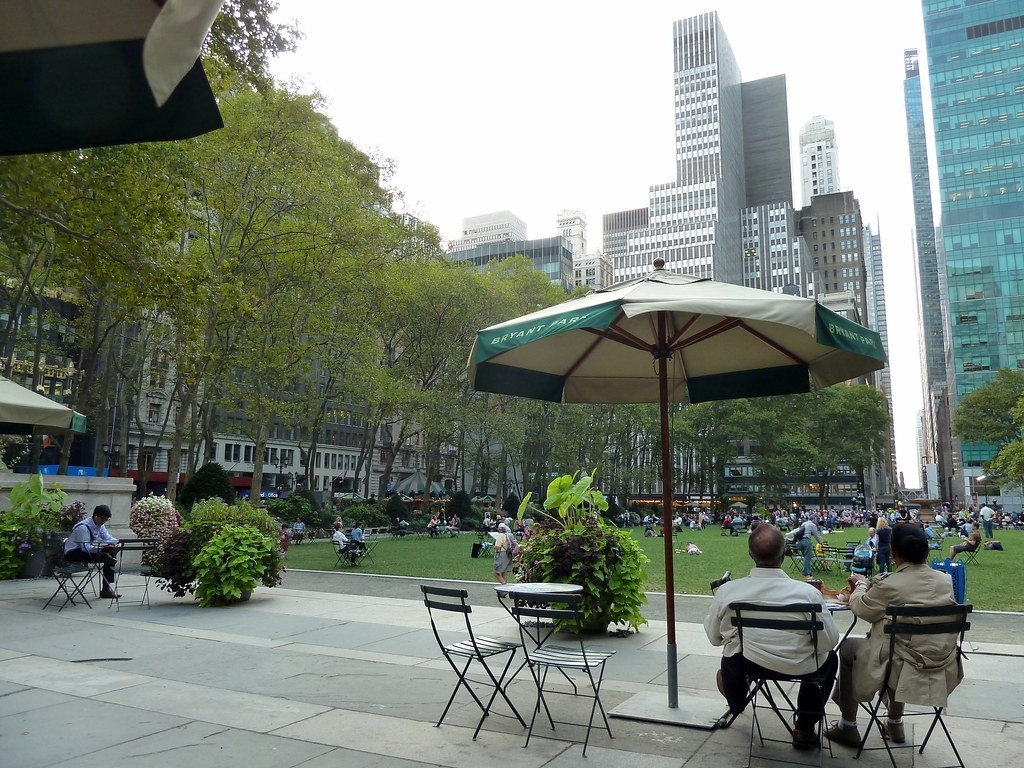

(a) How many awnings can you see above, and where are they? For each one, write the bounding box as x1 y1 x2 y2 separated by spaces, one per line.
2 4 225 157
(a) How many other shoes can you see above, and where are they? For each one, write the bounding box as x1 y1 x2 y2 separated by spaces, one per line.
880 721 904 743
823 719 861 748
716 668 726 697
792 728 820 750
100 589 122 598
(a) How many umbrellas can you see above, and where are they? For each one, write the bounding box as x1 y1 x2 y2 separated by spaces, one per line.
388 468 447 498
0 375 87 434
465 256 886 709
471 494 495 507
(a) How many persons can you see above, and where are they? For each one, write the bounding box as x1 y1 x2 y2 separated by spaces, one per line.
825 522 963 749
493 522 513 584
427 514 462 538
788 515 824 576
932 501 1024 534
482 513 539 530
701 523 841 750
394 518 406 536
63 505 122 599
276 517 308 552
408 506 424 521
622 503 929 536
332 517 367 568
863 517 893 572
947 521 982 558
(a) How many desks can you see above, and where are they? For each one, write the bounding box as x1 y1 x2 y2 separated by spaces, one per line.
358 540 378 565
491 584 584 695
73 539 119 599
793 604 891 741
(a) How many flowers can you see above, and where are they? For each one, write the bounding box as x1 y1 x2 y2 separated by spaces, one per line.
152 495 289 608
0 469 88 580
508 464 649 635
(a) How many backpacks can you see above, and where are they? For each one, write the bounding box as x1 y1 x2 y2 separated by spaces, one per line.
794 522 810 540
501 531 517 557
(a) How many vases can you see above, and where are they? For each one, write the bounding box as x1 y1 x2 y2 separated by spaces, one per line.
22 538 56 577
239 585 251 602
55 531 72 567
581 591 611 634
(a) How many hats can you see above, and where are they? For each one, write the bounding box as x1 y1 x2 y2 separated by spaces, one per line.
498 523 505 528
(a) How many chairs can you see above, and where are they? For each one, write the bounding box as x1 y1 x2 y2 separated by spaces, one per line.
476 533 495 558
331 531 378 568
307 528 317 545
671 527 681 548
710 570 973 768
720 520 861 574
419 584 618 758
41 537 159 612
387 522 457 542
926 530 981 567
